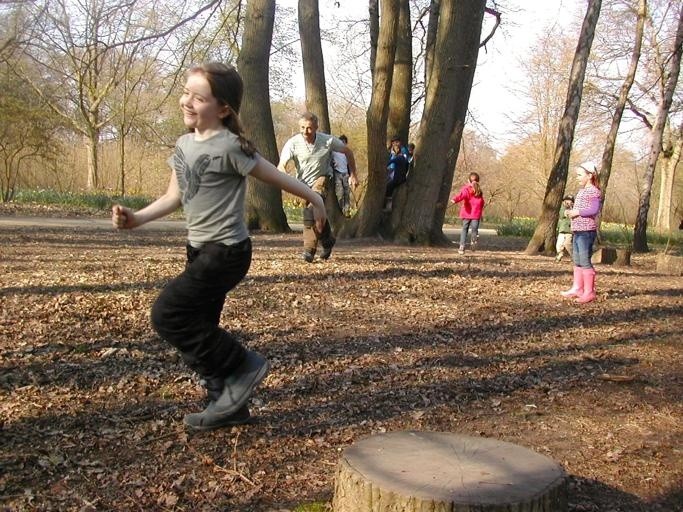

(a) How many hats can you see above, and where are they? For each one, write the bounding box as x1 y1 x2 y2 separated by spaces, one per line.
561 194 574 203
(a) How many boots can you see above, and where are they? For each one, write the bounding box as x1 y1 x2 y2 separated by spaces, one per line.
559 266 584 298
576 268 596 303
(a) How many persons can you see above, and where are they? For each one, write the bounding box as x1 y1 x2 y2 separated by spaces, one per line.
380 134 414 213
109 59 328 433
446 171 484 255
272 111 360 264
327 133 352 219
554 194 573 264
559 160 600 303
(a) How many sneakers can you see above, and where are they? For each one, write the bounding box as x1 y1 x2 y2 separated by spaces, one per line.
212 350 270 420
319 237 336 259
345 211 351 218
471 237 476 251
302 250 314 263
182 399 252 430
